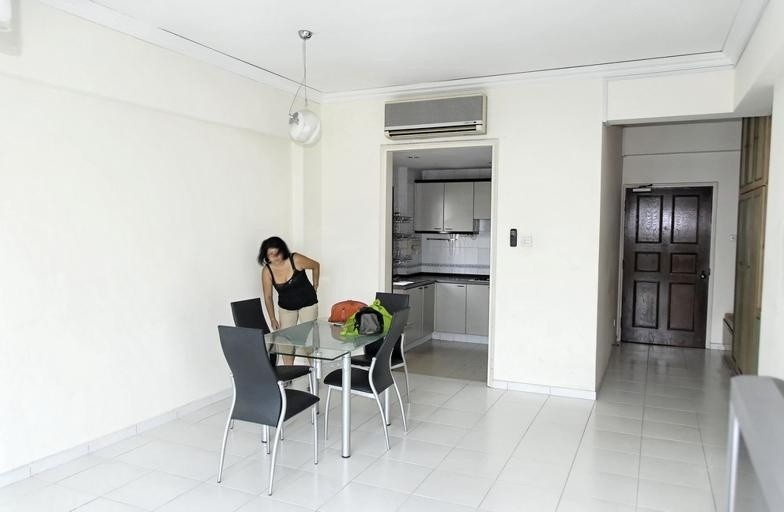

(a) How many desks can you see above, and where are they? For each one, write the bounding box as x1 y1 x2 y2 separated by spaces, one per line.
260 318 387 459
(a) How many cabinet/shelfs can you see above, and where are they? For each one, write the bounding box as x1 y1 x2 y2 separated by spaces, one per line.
393 220 413 268
412 180 480 236
434 283 489 336
393 284 434 349
472 181 491 221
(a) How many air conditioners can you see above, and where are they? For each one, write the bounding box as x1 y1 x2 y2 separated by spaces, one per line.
385 92 487 142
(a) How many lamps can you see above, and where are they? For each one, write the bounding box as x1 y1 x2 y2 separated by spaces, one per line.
288 30 324 146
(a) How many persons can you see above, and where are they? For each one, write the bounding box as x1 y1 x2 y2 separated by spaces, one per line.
259 236 320 393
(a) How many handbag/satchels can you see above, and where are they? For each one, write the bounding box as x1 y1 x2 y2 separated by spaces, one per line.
328 300 368 323
355 308 384 334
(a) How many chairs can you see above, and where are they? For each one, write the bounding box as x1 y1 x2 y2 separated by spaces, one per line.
323 306 411 451
350 291 412 404
230 296 317 442
216 323 323 495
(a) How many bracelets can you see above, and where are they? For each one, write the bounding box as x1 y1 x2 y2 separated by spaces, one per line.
270 317 276 320
